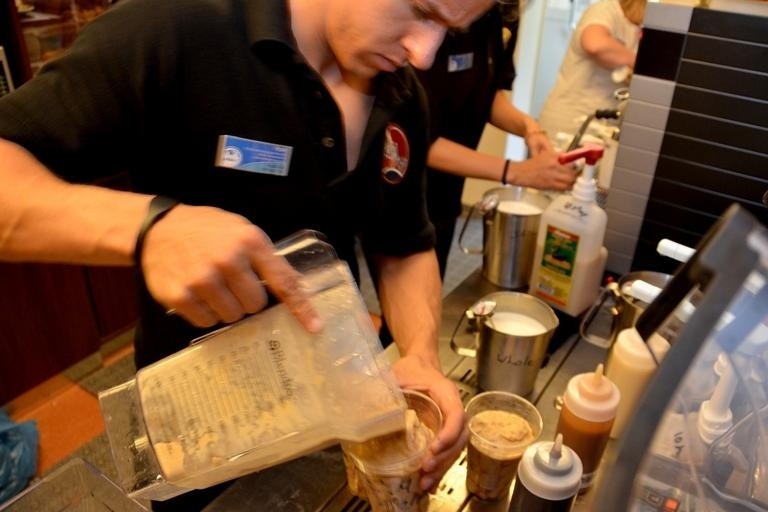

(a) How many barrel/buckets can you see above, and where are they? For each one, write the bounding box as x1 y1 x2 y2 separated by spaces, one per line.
460 187 556 285
448 288 564 397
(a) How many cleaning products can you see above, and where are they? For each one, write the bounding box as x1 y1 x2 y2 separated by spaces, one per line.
531 146 611 318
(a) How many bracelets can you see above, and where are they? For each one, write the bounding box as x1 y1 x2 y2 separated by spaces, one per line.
132 194 180 272
501 159 509 185
525 129 547 145
376 1 581 351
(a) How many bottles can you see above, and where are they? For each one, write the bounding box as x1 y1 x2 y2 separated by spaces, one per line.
605 322 671 439
553 363 622 497
507 433 583 512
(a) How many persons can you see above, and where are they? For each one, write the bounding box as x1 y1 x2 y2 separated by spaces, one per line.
537 1 647 157
0 0 500 512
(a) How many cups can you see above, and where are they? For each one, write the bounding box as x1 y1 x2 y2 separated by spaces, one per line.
463 391 543 505
339 388 444 512
